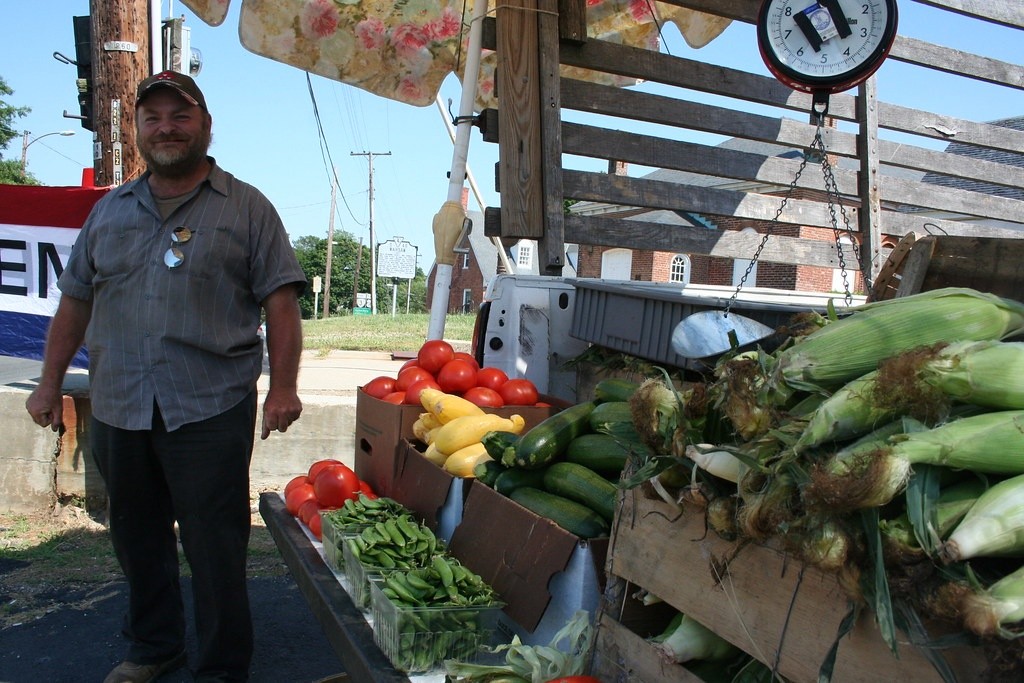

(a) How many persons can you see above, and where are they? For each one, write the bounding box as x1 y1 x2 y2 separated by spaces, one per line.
24 70 308 683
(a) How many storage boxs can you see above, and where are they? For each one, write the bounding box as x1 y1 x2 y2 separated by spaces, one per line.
338 530 415 608
353 386 571 502
363 572 505 670
392 437 473 537
446 479 608 655
316 507 362 573
563 276 850 376
592 460 1024 683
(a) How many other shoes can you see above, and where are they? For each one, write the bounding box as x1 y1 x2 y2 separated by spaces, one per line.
103 648 187 683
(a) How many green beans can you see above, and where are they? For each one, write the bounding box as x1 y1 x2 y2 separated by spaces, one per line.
321 490 505 675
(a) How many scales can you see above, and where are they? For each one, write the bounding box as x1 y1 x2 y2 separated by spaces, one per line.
673 0 899 360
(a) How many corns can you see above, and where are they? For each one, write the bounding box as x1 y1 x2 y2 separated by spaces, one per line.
636 288 1024 666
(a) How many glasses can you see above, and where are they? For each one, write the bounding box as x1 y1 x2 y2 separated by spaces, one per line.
164 226 196 271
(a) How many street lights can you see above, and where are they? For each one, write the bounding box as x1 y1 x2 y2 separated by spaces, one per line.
19 130 76 182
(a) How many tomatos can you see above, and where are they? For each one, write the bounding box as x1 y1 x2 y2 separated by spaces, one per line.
541 674 603 683
361 340 553 407
284 458 380 541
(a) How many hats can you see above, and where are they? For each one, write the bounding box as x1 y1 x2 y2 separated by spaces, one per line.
134 70 207 111
